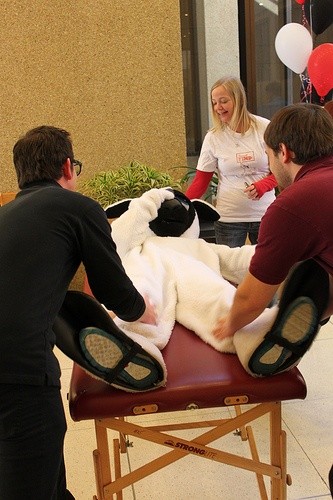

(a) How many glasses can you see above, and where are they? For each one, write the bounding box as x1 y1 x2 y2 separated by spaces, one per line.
64 158 81 176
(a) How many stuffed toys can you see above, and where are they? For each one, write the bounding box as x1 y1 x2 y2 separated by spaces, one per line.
54 186 329 393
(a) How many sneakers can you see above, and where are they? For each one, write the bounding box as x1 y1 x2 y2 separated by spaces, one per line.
248 296 317 377
79 327 160 388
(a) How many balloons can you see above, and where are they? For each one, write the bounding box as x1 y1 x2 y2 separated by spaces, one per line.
273 16 313 77
308 42 332 98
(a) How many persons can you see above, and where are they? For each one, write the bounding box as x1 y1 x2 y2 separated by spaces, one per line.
0 126 156 500
184 75 278 248
211 103 333 500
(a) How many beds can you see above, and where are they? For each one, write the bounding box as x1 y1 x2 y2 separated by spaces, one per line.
67 274 307 500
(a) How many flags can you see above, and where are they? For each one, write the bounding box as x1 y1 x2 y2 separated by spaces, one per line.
296 0 307 4
